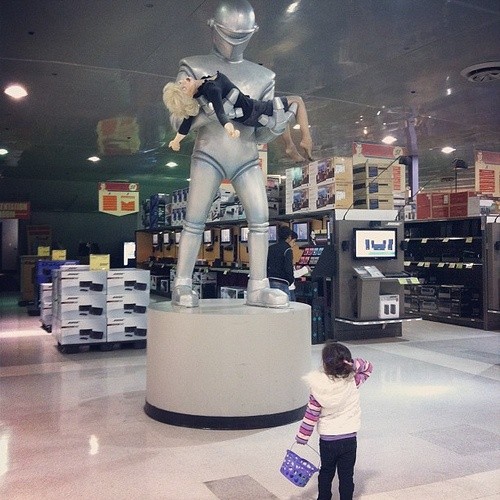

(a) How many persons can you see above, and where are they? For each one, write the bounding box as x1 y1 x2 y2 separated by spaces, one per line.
267 226 295 302
289 232 298 248
295 337 374 500
171 0 298 310
161 73 317 162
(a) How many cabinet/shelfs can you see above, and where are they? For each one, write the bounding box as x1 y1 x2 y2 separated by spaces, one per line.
404 215 488 330
134 209 404 344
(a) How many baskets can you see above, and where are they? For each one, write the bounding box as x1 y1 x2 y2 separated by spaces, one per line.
280 440 321 487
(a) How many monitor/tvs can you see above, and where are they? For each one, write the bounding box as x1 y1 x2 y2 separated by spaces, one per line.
163 233 169 243
203 229 212 243
220 228 232 243
175 231 181 244
239 226 249 242
352 227 397 260
152 233 158 244
292 221 310 241
269 224 280 241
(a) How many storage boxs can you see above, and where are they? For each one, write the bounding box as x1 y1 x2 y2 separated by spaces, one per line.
20 156 482 345
